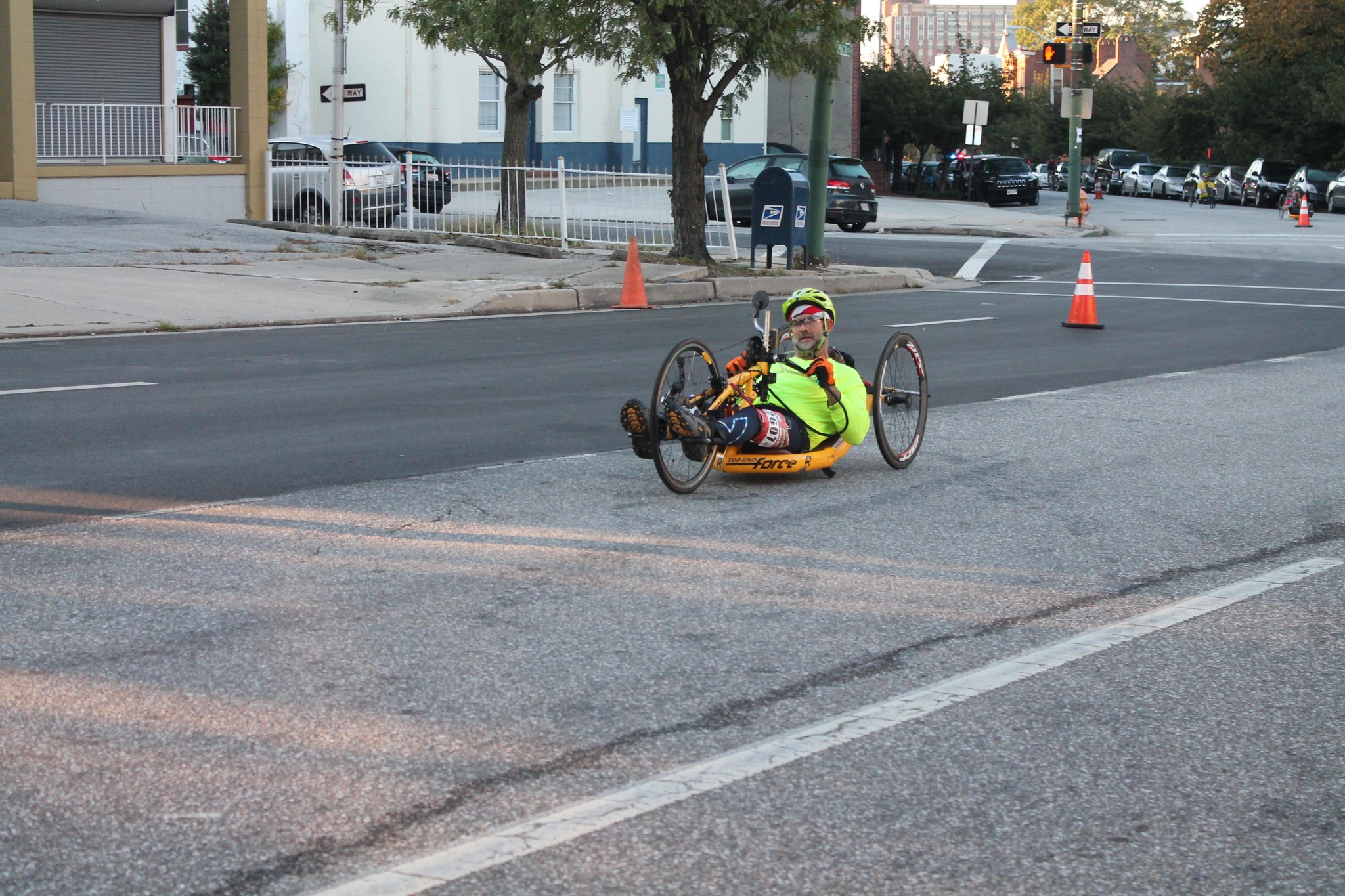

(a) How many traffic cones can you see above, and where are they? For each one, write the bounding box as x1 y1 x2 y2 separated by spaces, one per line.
607 236 659 310
1060 250 1105 331
1294 194 1314 228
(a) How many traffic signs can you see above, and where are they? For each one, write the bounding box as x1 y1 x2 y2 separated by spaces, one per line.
320 83 367 104
1056 21 1102 39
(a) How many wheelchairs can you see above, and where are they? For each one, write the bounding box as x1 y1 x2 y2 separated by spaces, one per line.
1188 184 1217 209
1277 194 1301 220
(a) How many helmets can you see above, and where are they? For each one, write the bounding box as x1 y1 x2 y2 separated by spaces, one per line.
781 288 836 325
1292 187 1300 192
1202 172 1210 177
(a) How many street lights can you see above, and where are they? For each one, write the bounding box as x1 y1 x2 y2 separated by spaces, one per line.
1004 26 1054 117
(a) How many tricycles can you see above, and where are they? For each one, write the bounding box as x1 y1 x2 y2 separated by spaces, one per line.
627 289 920 496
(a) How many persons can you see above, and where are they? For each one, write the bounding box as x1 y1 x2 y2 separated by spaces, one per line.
1282 187 1303 215
1046 155 1058 188
620 289 870 462
1196 172 1216 199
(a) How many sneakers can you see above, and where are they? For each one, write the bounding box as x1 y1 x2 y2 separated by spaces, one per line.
620 398 660 459
664 403 715 462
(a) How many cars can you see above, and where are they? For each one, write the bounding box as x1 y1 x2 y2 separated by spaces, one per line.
1213 165 1249 205
1031 162 1091 192
878 153 1040 206
703 152 879 233
1182 163 1227 202
1284 164 1341 208
1121 163 1162 197
177 132 232 164
1149 165 1192 200
267 135 407 228
383 144 453 214
1325 170 1345 213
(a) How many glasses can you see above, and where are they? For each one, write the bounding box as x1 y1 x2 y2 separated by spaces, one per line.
1203 176 1209 178
789 316 823 329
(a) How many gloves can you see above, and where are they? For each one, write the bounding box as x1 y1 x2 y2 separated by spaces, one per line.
805 357 836 386
1290 203 1294 207
725 351 748 378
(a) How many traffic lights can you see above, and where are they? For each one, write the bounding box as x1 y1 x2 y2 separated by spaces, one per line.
1042 42 1066 65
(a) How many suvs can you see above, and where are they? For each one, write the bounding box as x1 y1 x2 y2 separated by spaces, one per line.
1094 148 1151 195
1240 157 1301 208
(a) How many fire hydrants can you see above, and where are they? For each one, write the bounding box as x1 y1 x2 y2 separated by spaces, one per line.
1073 186 1092 226
